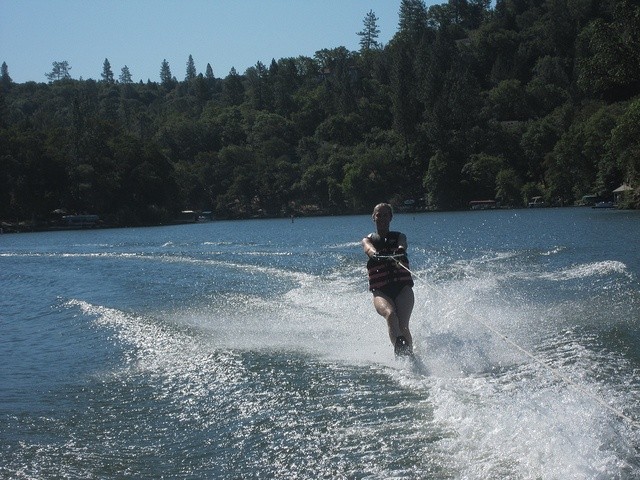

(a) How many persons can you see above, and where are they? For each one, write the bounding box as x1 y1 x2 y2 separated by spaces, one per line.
361 202 414 356
